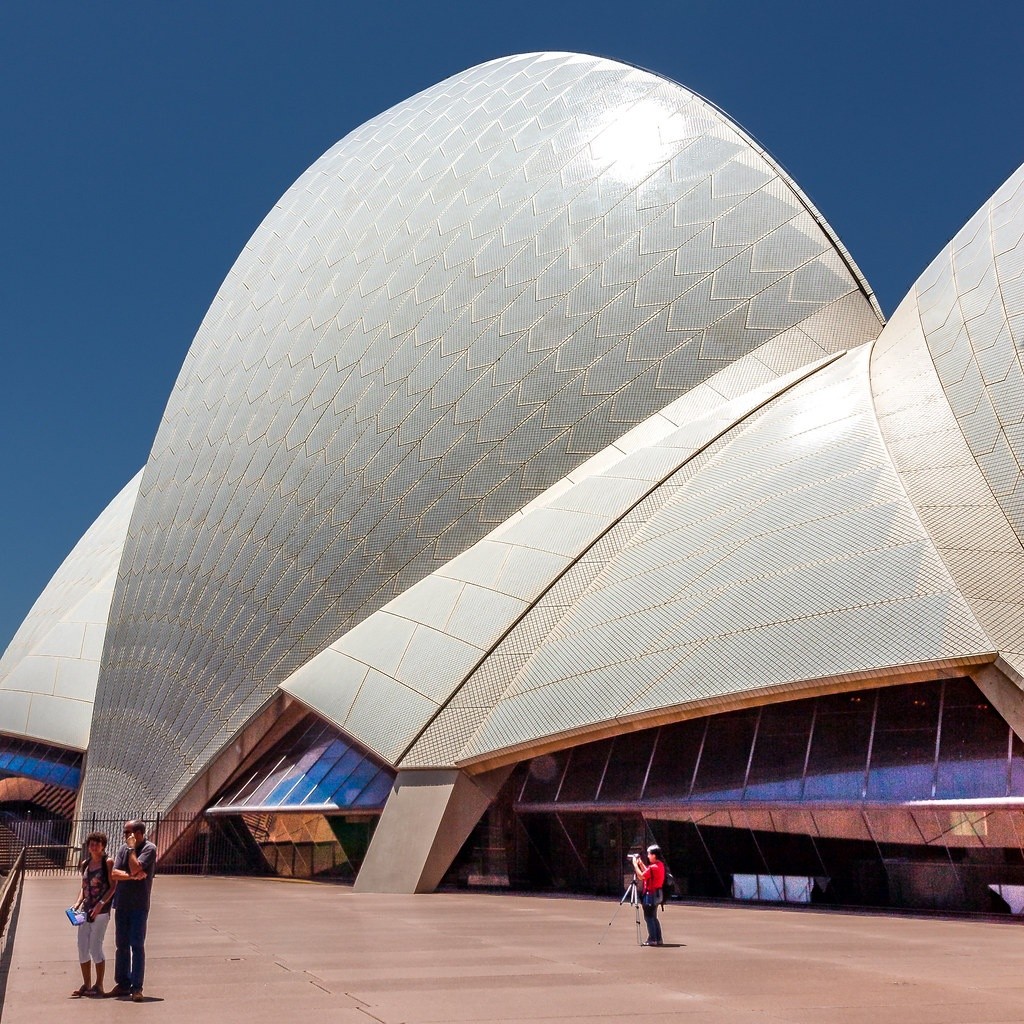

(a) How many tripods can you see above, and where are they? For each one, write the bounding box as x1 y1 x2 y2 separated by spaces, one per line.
598 874 643 947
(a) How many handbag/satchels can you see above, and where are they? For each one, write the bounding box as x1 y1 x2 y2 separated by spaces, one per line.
662 863 675 905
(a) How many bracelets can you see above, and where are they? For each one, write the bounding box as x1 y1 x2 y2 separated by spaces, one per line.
99 899 105 905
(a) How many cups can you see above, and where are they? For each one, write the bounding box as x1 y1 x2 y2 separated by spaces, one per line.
646 892 652 904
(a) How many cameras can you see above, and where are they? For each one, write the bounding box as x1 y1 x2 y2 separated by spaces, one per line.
627 854 640 862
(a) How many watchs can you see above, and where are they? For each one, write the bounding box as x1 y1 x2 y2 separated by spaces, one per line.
126 847 135 853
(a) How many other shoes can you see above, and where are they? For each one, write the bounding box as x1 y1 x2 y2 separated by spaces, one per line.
132 989 144 1001
74 985 91 995
86 985 104 995
643 939 664 946
104 985 130 997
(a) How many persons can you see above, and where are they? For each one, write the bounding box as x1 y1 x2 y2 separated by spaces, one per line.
633 845 665 946
102 819 157 1001
70 832 118 998
